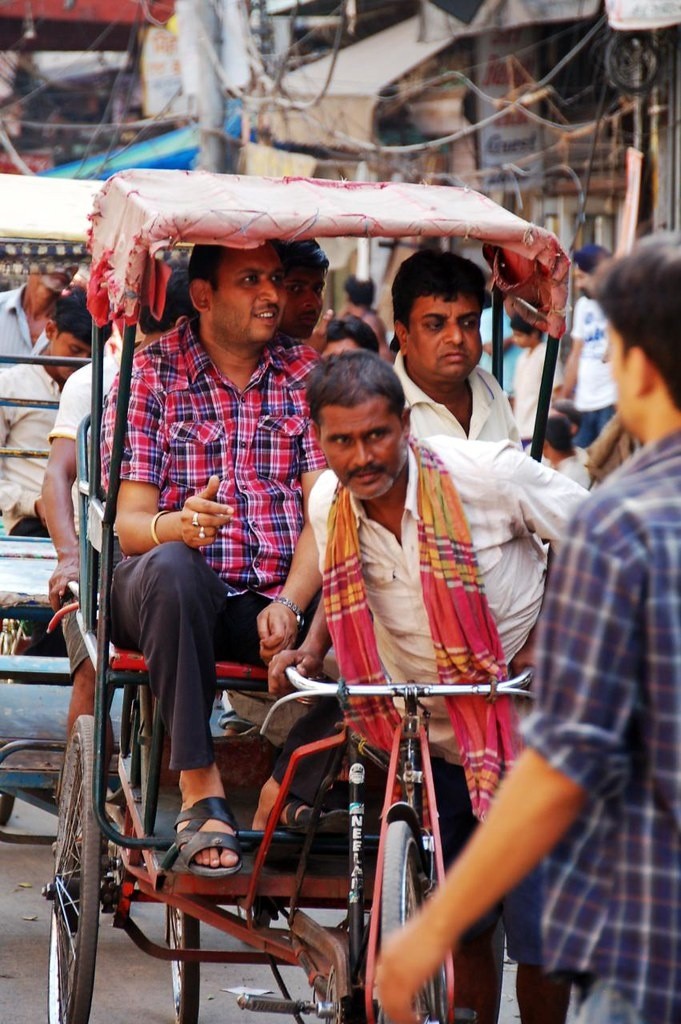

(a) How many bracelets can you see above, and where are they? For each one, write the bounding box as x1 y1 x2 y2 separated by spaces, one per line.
152 510 170 546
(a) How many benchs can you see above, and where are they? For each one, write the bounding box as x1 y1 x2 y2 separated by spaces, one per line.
75 610 270 692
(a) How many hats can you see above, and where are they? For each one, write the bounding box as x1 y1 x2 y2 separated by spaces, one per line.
572 242 612 273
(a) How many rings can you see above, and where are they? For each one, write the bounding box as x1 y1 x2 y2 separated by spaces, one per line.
199 527 205 539
192 512 199 527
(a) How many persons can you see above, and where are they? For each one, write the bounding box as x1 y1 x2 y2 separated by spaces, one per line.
271 237 645 492
0 254 82 369
313 249 522 834
98 238 327 877
0 283 112 683
375 230 681 1024
269 352 591 1024
41 258 198 856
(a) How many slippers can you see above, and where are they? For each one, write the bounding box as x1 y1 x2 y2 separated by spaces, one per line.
267 794 349 834
173 796 243 877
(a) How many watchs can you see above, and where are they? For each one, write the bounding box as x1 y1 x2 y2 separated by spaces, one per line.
273 596 305 631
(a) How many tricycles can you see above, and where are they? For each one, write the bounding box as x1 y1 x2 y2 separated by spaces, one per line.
47 171 565 1024
0 179 119 825
